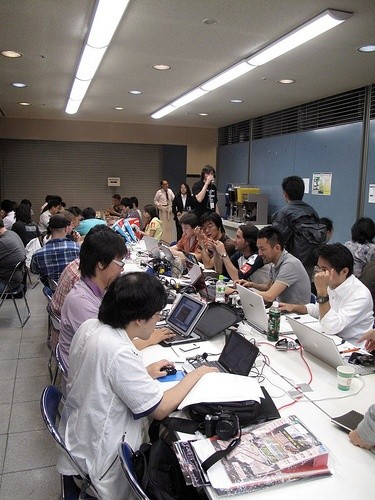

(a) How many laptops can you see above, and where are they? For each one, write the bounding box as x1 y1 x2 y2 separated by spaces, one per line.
144 236 165 259
237 284 295 336
287 314 375 379
162 245 180 265
194 301 240 340
184 330 258 376
154 293 208 345
187 253 222 281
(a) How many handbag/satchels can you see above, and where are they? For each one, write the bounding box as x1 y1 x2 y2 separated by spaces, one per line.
137 438 200 500
188 400 260 424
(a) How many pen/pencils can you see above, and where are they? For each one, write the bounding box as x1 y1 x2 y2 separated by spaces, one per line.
285 316 301 320
338 348 362 354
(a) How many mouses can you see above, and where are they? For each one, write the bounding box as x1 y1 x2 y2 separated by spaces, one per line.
160 368 177 375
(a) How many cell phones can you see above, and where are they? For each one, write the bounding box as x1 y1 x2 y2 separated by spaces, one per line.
180 344 200 352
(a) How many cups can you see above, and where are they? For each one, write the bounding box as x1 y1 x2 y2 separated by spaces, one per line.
337 365 355 391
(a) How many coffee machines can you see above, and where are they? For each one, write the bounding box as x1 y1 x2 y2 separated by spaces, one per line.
222 183 256 223
236 186 269 225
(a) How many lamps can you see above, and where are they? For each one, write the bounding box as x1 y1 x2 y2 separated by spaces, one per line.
64 0 131 116
150 10 354 119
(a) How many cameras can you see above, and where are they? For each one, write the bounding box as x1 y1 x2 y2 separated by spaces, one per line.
201 409 239 442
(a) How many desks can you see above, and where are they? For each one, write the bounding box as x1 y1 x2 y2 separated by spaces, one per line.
230 303 375 451
98 260 375 499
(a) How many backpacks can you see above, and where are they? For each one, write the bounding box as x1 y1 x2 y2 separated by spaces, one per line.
293 218 328 270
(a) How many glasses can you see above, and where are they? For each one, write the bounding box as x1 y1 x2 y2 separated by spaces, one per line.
112 260 127 267
314 266 329 274
202 225 216 231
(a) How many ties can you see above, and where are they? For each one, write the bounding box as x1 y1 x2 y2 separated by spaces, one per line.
164 189 169 200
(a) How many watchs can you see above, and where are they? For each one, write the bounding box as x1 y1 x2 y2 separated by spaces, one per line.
315 294 329 304
220 252 228 258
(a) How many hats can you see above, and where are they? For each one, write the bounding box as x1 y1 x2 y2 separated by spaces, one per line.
49 214 71 228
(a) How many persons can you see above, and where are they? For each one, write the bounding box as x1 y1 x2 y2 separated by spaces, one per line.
12 204 31 247
168 212 204 262
0 199 16 230
153 179 175 219
48 224 116 348
225 226 311 306
276 241 374 353
172 183 194 242
192 165 221 218
194 212 238 269
30 214 81 294
270 175 320 282
206 223 275 285
138 204 163 242
0 216 26 293
43 209 80 245
56 271 220 500
343 216 375 316
20 195 142 229
349 326 374 449
59 230 176 400
320 217 334 245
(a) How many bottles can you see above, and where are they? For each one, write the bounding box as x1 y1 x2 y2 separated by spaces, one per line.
267 301 281 342
215 275 226 303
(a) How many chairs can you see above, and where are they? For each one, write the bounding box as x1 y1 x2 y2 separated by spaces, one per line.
118 442 150 500
25 238 42 287
41 385 102 500
43 287 61 351
0 262 32 327
47 306 70 387
